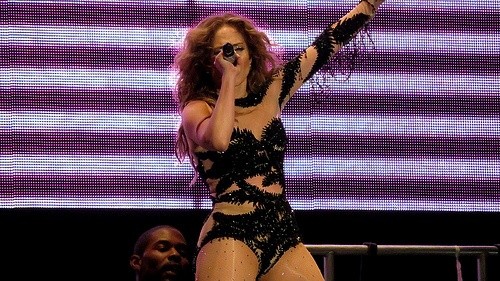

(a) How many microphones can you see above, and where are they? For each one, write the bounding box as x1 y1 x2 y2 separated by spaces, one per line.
222 42 238 67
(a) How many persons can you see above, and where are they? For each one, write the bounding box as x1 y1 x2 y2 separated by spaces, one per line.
170 0 386 281
129 225 189 281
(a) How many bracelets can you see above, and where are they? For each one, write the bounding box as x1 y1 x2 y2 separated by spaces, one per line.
362 0 378 13
